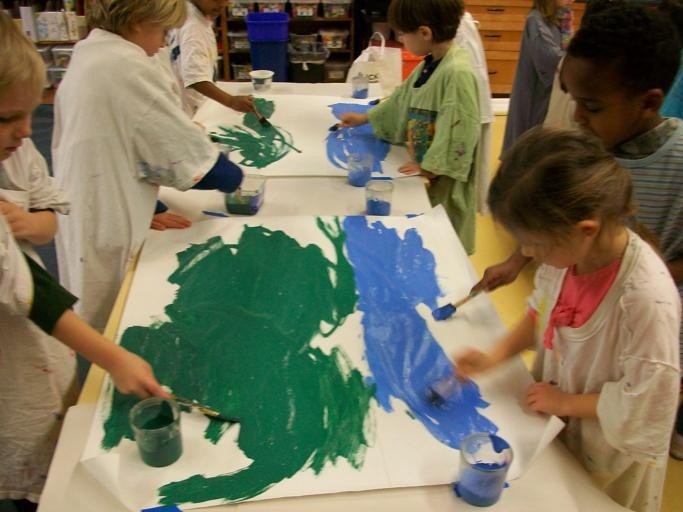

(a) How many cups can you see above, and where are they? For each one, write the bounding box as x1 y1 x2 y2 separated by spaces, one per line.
348 153 394 216
352 76 370 99
128 397 184 467
457 432 513 507
250 70 275 91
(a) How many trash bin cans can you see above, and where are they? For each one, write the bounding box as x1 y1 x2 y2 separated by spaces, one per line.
247 12 289 82
289 43 327 82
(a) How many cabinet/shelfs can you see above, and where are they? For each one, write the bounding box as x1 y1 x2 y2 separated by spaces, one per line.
460 0 586 98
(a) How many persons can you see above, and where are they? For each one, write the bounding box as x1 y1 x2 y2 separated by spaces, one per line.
452 123 683 511
157 0 255 122
333 0 482 255
500 0 575 162
50 1 246 332
0 10 175 512
470 12 683 295
454 0 495 217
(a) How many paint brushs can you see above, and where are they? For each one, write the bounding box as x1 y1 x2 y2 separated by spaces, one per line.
169 394 241 423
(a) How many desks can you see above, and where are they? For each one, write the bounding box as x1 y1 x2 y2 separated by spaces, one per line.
35 81 637 512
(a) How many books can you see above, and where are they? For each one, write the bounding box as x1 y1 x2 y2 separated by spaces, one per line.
14 6 89 41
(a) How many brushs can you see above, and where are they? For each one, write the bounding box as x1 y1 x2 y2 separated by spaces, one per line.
254 110 271 128
329 123 346 131
424 374 459 408
432 289 484 321
